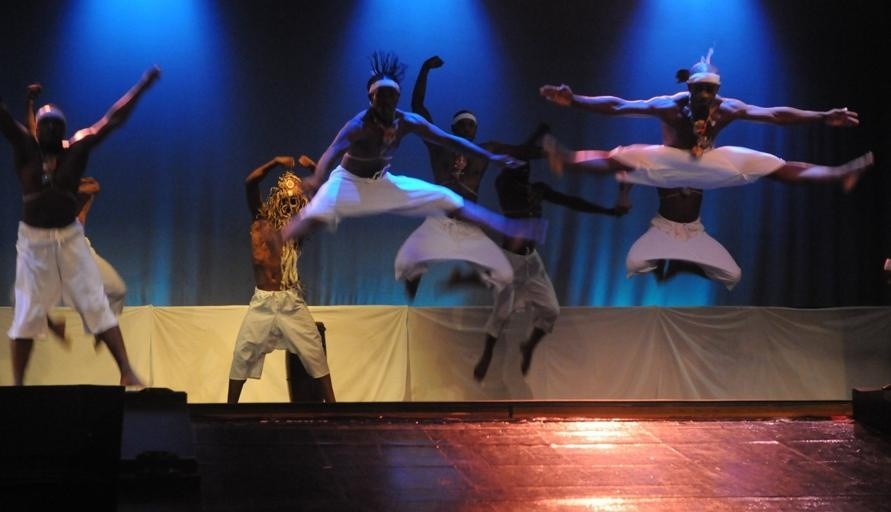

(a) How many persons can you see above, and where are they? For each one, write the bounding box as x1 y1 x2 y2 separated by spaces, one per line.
474 121 616 381
263 50 550 260
615 182 743 293
27 83 127 350
228 155 335 404
0 64 163 387
538 47 876 194
394 56 546 300
